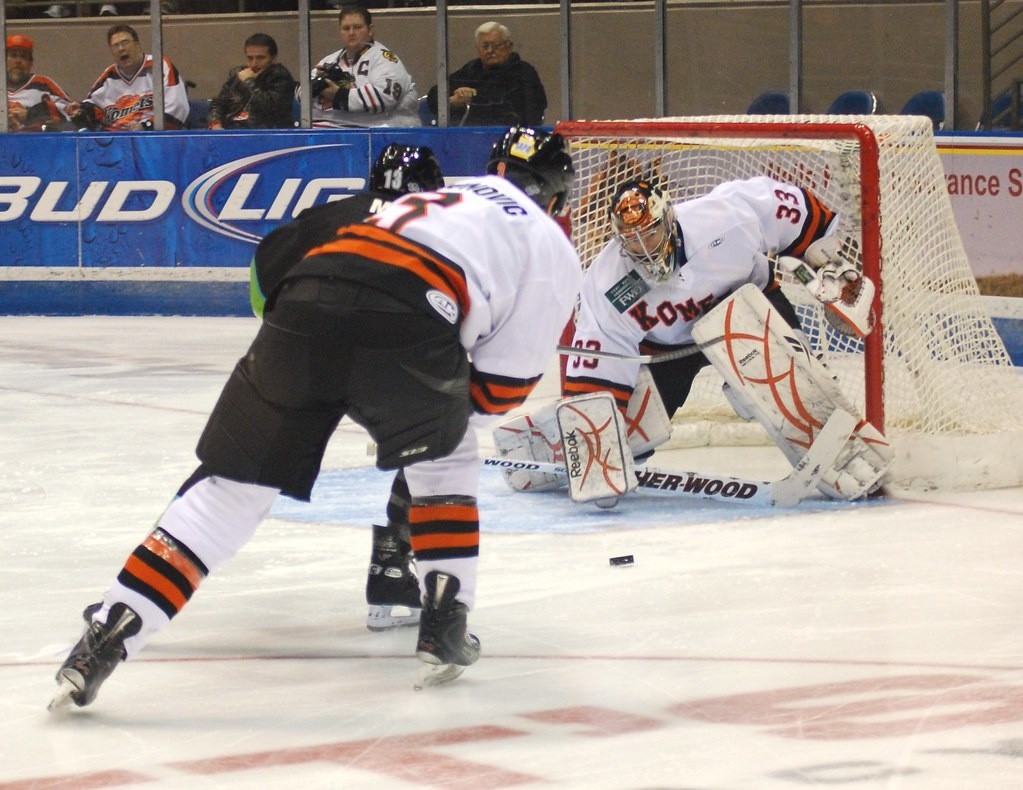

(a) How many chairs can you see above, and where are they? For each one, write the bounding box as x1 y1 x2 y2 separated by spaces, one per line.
746 90 790 113
899 90 945 122
187 99 210 128
417 95 431 123
826 90 879 115
975 90 1023 131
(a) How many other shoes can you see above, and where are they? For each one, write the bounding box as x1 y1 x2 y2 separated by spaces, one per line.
99 4 118 16
44 4 72 18
142 0 181 15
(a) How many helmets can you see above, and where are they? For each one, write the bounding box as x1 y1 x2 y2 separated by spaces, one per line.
488 121 574 218
609 180 674 279
368 143 445 200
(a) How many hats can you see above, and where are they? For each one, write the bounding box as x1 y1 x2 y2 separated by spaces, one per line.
6 34 34 50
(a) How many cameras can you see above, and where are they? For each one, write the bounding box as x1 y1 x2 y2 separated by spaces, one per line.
311 62 351 99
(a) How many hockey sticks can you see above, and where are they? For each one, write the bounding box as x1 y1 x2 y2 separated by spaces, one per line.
363 406 858 510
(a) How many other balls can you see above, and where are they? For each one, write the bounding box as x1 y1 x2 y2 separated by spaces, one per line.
608 554 636 567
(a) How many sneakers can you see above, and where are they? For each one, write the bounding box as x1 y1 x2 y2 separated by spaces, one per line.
366 524 424 631
413 570 480 692
46 602 142 713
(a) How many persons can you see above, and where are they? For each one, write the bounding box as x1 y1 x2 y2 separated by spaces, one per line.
492 176 896 509
207 32 295 129
247 144 444 631
294 5 422 127
67 24 189 131
6 33 73 132
426 21 547 126
46 125 584 710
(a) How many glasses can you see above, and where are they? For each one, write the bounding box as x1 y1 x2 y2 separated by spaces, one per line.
480 41 509 50
